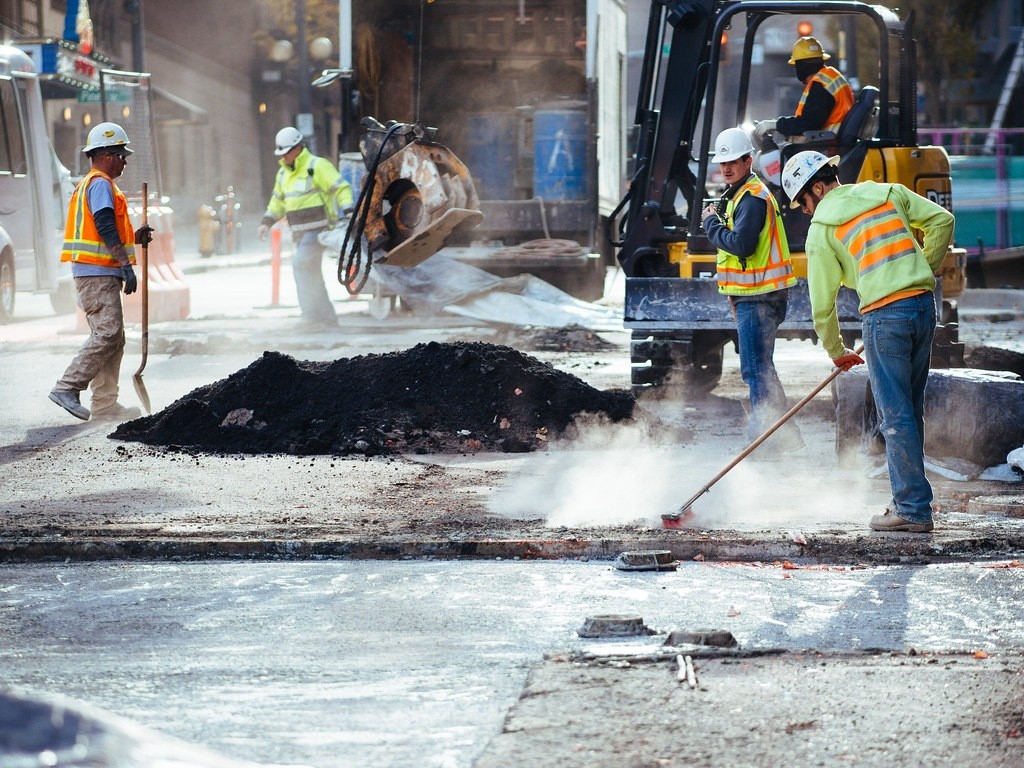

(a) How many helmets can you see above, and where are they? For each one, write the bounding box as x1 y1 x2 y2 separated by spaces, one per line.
710 127 755 163
273 127 304 155
788 36 831 65
781 150 840 203
82 122 130 152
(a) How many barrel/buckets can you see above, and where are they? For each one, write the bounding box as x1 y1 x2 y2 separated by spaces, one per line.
466 109 518 201
338 152 368 220
530 95 588 202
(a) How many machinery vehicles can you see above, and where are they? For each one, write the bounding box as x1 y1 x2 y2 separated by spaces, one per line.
603 1 970 401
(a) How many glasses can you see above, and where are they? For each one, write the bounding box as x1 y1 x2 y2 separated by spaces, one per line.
797 173 831 206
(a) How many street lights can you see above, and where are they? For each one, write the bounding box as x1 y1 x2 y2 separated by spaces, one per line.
312 67 352 87
271 34 333 156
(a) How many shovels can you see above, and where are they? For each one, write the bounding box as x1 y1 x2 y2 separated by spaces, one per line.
132 182 151 415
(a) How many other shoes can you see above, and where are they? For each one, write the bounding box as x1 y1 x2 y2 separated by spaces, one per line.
48 391 90 420
868 501 934 533
91 401 142 420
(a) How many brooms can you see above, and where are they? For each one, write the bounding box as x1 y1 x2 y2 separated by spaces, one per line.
660 344 865 530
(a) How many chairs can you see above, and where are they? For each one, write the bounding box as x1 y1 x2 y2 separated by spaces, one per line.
783 85 878 154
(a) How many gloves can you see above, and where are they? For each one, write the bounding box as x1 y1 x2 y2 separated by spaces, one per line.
832 347 865 372
134 225 154 244
120 264 136 295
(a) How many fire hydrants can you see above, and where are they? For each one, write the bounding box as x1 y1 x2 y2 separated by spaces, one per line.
195 204 219 258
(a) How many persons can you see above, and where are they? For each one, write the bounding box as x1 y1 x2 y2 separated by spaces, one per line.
751 36 852 208
258 127 356 326
701 127 807 464
45 123 155 422
781 150 957 532
